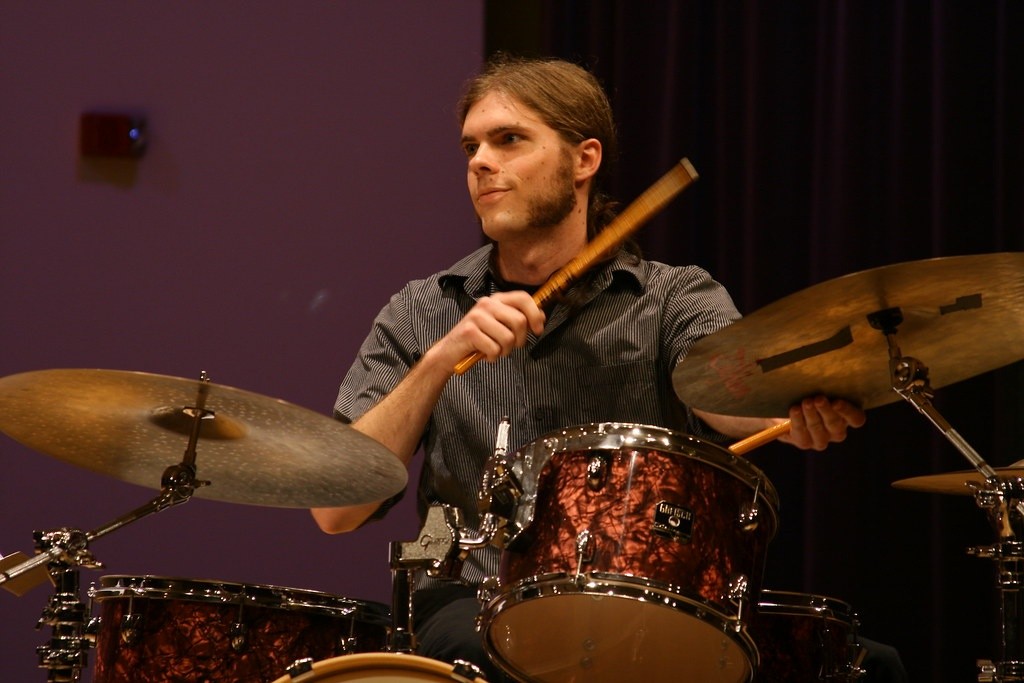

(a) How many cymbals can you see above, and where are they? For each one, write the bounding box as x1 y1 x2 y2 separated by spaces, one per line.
891 466 1024 497
672 252 1024 418
0 368 409 509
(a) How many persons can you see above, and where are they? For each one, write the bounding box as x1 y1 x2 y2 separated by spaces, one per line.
309 61 869 683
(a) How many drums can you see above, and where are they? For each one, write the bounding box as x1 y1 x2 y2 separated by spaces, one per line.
758 583 861 683
92 574 393 683
473 421 780 683
271 652 489 683
